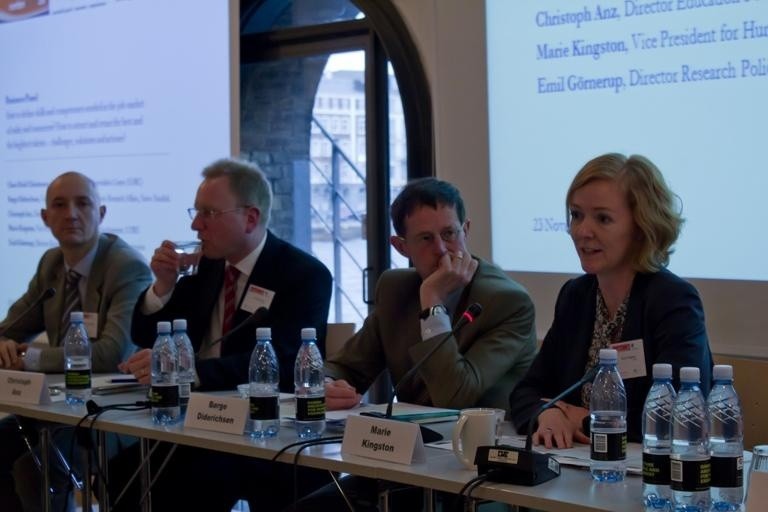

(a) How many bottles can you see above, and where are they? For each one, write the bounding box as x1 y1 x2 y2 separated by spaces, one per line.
295 328 326 439
150 322 181 425
250 329 280 437
65 312 91 407
171 319 195 419
708 365 744 512
671 367 711 511
642 363 677 512
590 348 626 482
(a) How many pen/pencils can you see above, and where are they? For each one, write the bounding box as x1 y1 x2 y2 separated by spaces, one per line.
104 378 138 383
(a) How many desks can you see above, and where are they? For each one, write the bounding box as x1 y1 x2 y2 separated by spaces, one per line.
0 369 768 512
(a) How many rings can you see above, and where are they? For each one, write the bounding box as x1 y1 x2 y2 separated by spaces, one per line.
543 426 553 433
453 254 462 260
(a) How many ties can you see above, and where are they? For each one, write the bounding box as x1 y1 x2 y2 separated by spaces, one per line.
222 266 241 335
56 271 81 347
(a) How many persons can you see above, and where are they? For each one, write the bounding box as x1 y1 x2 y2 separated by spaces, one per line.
0 172 153 511
508 153 715 451
322 180 540 512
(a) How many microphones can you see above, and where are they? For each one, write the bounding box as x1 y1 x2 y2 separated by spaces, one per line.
360 303 482 445
0 287 56 338
192 305 269 364
474 366 599 488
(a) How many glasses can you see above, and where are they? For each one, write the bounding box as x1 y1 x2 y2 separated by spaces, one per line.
400 224 466 250
187 204 251 224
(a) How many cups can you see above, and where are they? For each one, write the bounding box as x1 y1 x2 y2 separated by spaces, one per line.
452 408 496 472
491 409 506 442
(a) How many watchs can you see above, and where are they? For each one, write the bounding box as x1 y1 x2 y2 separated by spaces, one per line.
418 304 449 318
94 158 332 512
19 350 27 366
